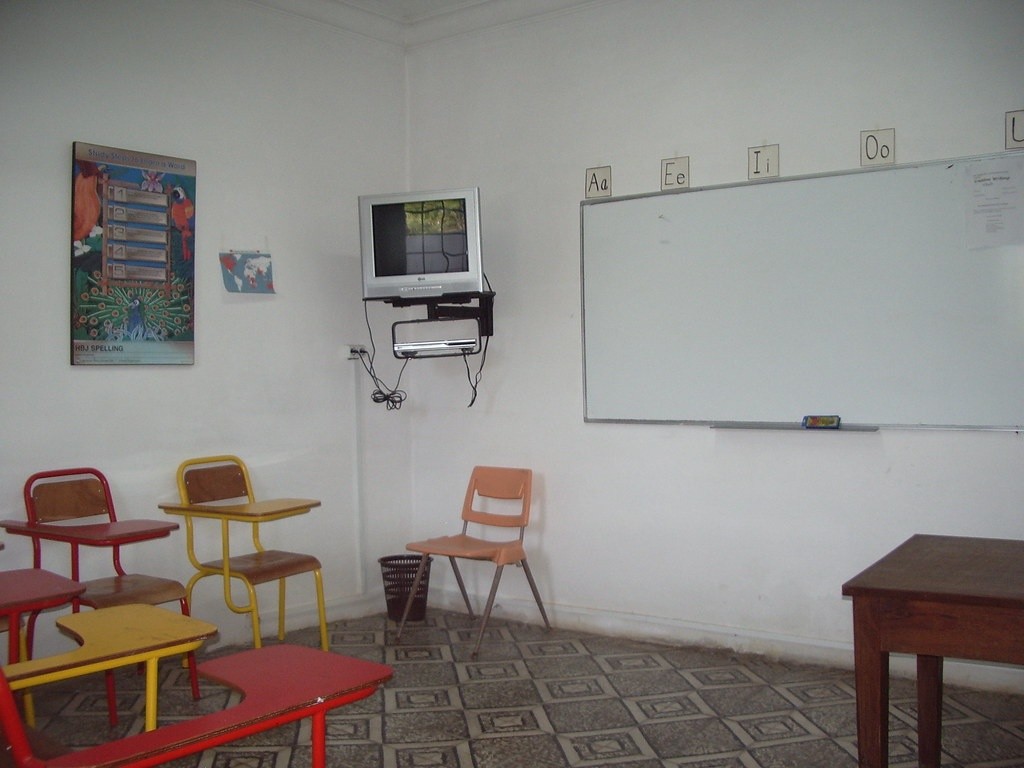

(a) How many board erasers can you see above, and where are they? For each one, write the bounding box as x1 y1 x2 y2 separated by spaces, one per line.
802 416 841 428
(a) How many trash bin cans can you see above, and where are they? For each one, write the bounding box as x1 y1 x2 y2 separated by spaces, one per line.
378 555 435 622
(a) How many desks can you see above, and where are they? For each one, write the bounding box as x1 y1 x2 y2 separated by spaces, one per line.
0 519 179 548
1 604 218 692
0 643 394 768
158 498 321 522
841 533 1024 768
0 568 86 616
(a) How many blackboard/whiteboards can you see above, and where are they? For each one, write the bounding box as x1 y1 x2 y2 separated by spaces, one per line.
579 150 1023 432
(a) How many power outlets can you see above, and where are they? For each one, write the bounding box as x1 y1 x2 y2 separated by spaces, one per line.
345 345 367 360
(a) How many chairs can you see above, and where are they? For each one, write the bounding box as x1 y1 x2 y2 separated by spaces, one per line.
24 467 200 728
177 455 328 669
395 466 552 658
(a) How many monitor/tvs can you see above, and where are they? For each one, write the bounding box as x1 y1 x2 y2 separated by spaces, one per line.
358 188 483 299
